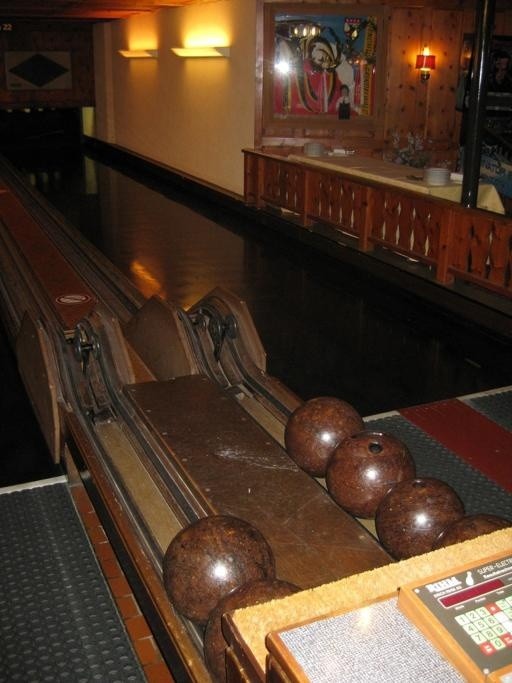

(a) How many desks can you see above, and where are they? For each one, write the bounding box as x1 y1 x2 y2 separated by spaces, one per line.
285 150 504 263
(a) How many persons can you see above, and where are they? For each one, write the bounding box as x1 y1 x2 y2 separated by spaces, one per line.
455 51 512 199
336 85 351 120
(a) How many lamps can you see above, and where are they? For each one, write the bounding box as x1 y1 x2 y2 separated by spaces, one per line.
414 54 436 81
